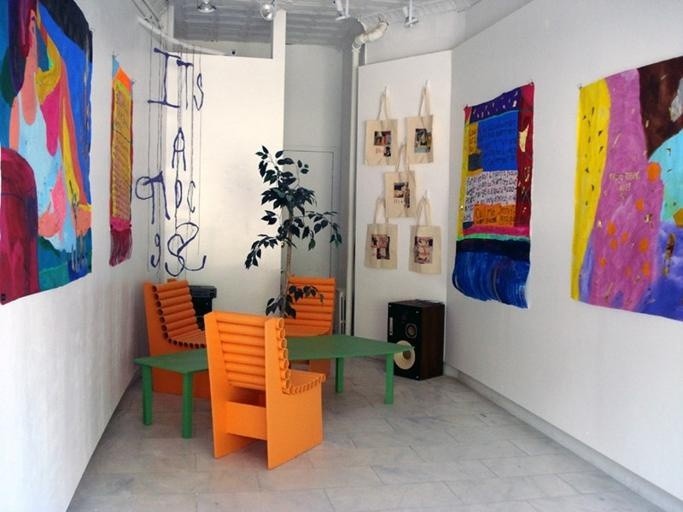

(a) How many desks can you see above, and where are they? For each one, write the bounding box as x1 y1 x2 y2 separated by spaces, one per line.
136 332 414 436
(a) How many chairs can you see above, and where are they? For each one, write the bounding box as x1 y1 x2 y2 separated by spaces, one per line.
148 275 338 468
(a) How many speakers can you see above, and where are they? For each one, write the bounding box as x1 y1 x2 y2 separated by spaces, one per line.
387 298 447 382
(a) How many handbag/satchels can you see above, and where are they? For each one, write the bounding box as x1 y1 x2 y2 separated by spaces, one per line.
365 118 399 165
365 225 398 268
406 115 433 163
408 225 440 274
385 171 416 217
